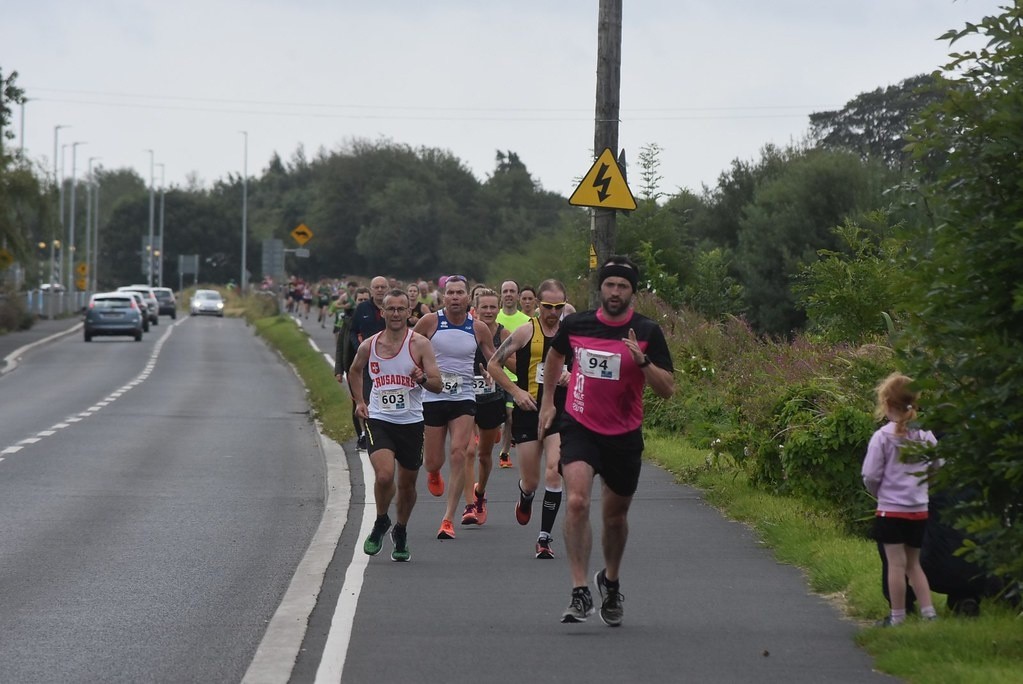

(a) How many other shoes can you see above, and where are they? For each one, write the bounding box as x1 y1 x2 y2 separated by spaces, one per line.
355 436 366 452
321 325 326 328
495 430 501 443
928 615 936 621
871 604 918 628
511 440 516 448
959 598 979 617
475 433 480 446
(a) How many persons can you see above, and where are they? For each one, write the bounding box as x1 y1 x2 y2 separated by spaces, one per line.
260 273 576 563
862 373 937 627
871 402 1002 626
536 257 674 627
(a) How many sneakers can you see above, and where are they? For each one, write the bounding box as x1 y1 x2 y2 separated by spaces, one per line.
364 516 392 556
390 523 412 561
498 450 513 468
461 503 478 524
560 590 595 623
535 535 555 559
473 482 488 525
594 568 625 627
427 469 445 497
436 519 456 539
515 479 536 527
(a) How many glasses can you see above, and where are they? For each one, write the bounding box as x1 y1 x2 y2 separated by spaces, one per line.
445 275 468 283
540 301 566 310
381 307 407 314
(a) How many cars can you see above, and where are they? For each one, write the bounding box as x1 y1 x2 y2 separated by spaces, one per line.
83 293 143 340
119 291 150 333
151 287 178 319
40 284 66 292
115 287 160 326
189 289 226 317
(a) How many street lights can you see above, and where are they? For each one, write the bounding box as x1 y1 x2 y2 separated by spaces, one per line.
153 162 166 287
142 148 155 290
236 130 247 303
50 124 99 320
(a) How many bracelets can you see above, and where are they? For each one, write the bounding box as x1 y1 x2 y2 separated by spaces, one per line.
417 373 427 388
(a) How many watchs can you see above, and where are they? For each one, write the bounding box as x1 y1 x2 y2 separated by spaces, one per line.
639 354 651 368
